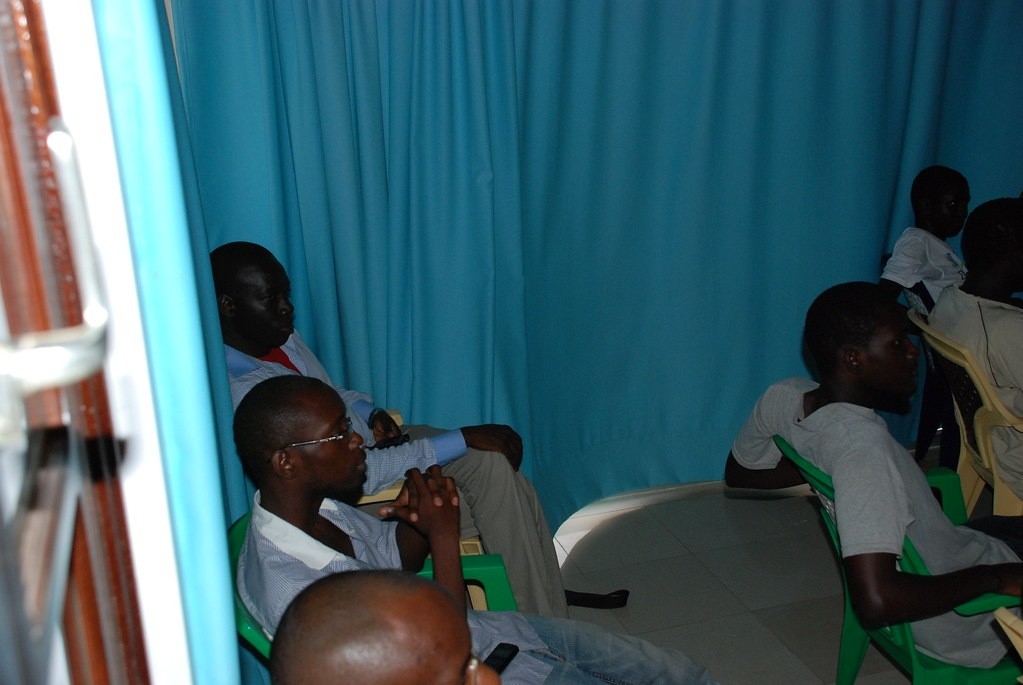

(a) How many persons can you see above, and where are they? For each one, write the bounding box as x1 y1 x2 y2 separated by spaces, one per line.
878 165 971 335
724 281 1022 671
211 240 573 621
235 374 713 685
268 570 503 684
927 197 1023 502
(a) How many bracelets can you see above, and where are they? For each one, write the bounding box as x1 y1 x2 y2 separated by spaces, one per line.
367 407 387 429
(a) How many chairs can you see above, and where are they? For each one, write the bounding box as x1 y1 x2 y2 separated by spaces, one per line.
228 408 517 669
885 253 962 466
907 306 1023 519
772 435 1023 685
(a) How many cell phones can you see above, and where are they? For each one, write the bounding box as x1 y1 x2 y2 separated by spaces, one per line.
369 435 409 451
483 643 519 675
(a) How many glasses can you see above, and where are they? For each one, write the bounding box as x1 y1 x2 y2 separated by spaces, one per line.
455 640 480 685
265 417 354 465
